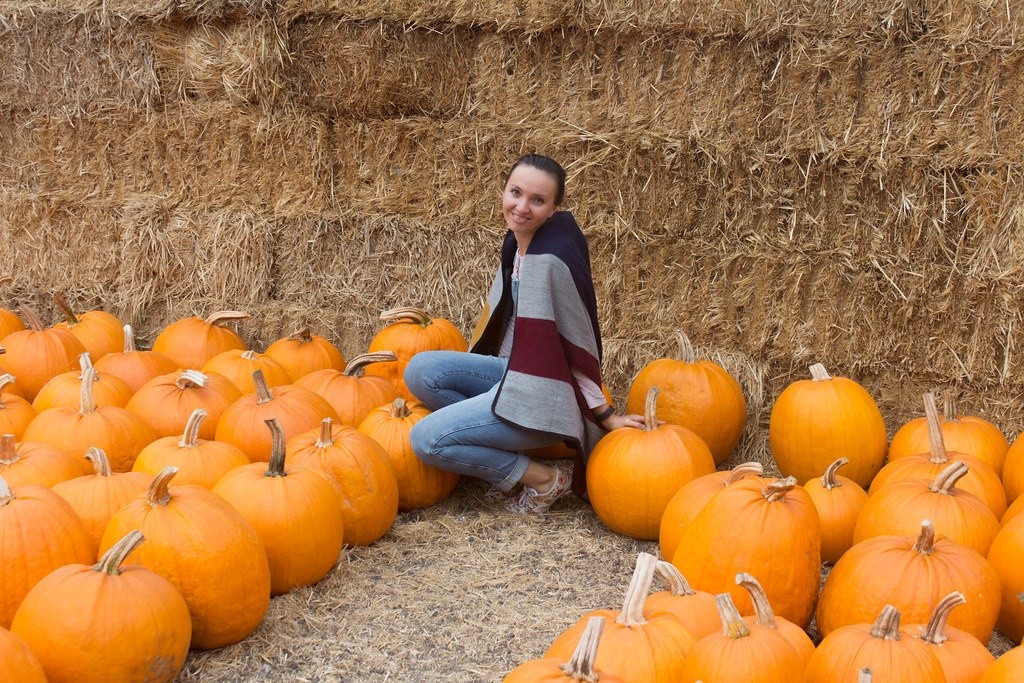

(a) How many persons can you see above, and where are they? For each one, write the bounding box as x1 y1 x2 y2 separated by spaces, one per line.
404 155 666 515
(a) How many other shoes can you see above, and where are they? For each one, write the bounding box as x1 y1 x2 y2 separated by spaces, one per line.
503 465 574 516
485 482 524 504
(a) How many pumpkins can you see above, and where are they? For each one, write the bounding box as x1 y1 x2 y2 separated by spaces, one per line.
0 287 465 683
503 331 1024 683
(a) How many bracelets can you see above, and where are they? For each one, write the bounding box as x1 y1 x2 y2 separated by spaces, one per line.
596 405 615 422
